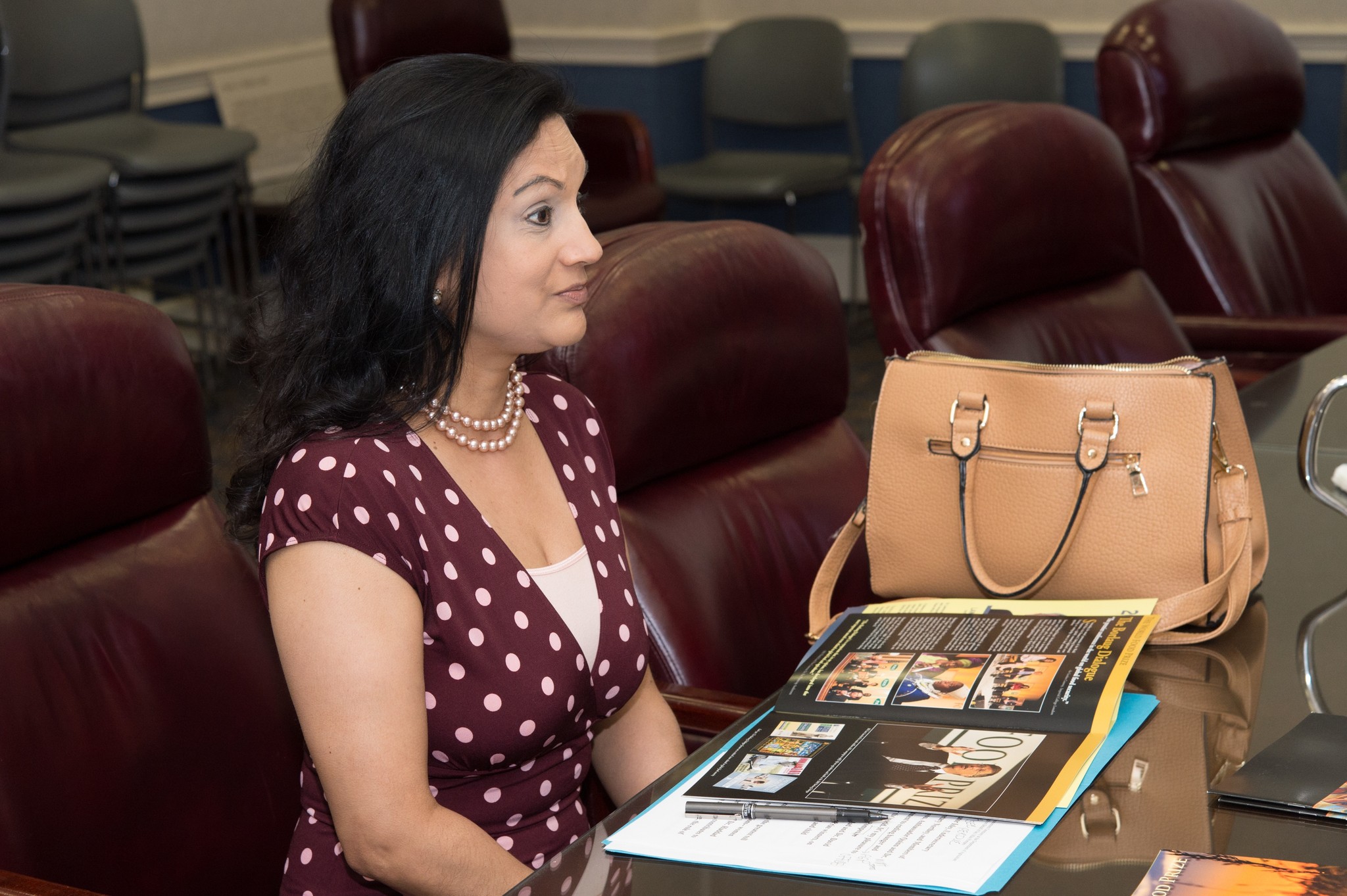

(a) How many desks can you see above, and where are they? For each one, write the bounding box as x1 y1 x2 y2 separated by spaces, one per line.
503 312 1347 896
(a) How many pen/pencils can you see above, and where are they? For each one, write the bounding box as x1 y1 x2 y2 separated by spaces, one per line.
685 801 889 823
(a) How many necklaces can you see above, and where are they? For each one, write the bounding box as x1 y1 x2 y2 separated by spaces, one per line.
391 361 527 454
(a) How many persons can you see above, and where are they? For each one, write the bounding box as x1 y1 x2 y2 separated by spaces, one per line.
821 648 1056 705
205 54 688 896
812 739 1003 803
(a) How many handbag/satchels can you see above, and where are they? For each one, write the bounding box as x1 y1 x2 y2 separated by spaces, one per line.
1030 594 1271 872
806 350 1269 644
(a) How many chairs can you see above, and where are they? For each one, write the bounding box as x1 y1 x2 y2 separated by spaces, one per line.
0 0 1347 896
